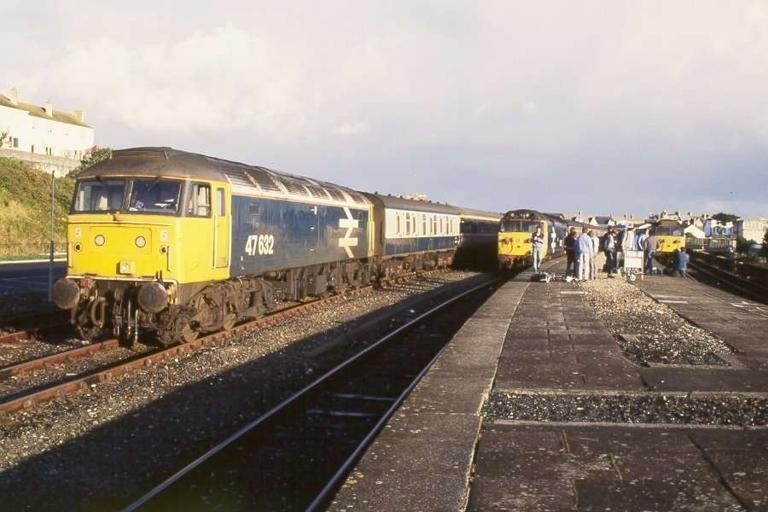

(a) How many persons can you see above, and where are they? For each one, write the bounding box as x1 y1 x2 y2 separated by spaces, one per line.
675 247 690 279
564 226 660 282
531 225 543 273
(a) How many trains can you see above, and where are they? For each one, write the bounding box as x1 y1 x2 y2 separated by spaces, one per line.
632 216 685 261
51 149 507 346
493 211 609 276
